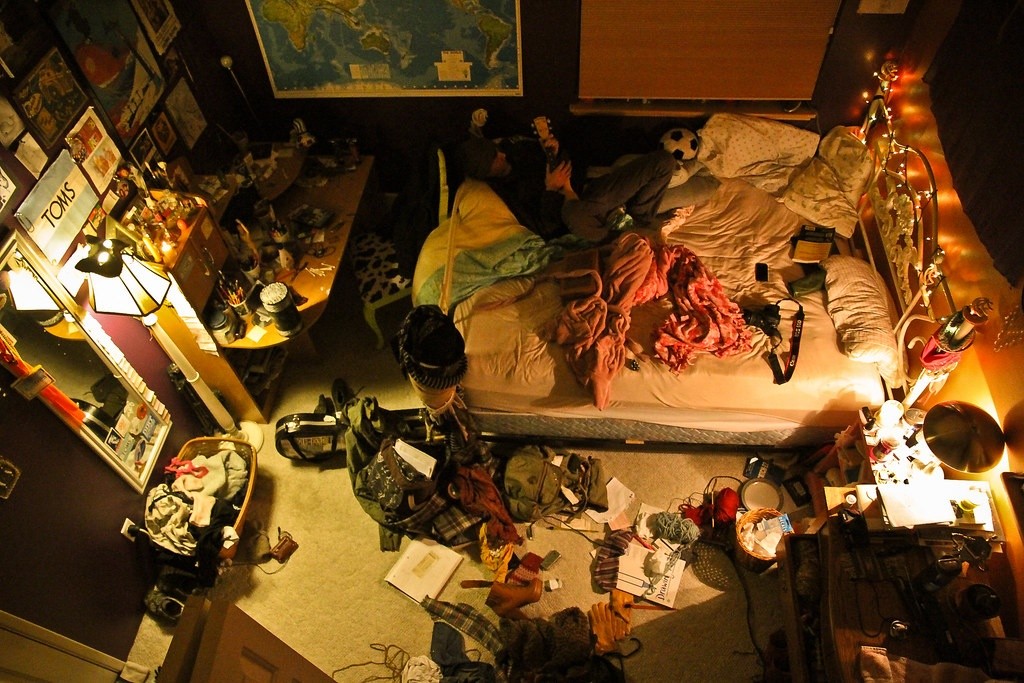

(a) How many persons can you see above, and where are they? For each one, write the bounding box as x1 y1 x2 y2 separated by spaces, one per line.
462 136 675 240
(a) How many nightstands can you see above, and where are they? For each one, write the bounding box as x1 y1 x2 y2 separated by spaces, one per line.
807 411 947 521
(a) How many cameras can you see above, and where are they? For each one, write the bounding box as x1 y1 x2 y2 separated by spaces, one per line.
744 304 781 336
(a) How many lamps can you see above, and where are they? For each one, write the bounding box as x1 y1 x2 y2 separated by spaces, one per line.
904 400 1006 485
74 234 264 453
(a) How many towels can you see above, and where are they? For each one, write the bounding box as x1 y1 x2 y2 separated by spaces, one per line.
857 645 1020 683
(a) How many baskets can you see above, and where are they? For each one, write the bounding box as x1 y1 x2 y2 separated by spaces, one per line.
153 436 258 558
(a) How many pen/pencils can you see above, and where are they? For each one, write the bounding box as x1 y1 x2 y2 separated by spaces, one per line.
219 275 245 304
269 220 288 237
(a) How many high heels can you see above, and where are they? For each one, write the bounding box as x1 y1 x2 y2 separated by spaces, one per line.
484 578 542 621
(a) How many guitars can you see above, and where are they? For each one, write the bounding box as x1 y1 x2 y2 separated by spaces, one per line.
529 114 612 243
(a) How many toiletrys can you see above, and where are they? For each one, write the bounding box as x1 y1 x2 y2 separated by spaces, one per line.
125 189 196 263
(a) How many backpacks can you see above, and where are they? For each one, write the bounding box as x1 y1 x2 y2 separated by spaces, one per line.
505 445 608 540
351 434 446 528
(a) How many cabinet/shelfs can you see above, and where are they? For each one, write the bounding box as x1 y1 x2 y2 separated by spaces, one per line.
776 482 1024 683
116 189 285 425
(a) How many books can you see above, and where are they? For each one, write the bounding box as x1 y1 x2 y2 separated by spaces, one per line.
616 542 686 608
792 225 835 263
384 535 464 604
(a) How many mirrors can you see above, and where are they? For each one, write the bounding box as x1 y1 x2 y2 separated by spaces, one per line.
0 228 174 495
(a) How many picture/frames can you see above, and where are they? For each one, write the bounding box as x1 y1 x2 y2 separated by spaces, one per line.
6 46 90 149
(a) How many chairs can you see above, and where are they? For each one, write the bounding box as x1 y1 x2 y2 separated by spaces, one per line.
349 148 449 354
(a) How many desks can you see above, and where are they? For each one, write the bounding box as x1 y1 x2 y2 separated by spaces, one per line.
211 154 375 369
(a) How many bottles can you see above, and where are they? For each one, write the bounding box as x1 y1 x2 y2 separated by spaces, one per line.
260 282 304 337
278 246 295 271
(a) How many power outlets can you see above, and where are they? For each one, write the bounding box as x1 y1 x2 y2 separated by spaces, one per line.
121 518 137 542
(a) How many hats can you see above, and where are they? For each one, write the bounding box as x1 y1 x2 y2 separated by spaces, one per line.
390 304 469 390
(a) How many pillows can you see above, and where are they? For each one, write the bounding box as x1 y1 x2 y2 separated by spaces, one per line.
696 111 820 197
774 126 874 237
818 254 907 388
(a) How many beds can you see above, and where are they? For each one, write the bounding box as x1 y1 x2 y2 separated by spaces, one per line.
407 60 994 448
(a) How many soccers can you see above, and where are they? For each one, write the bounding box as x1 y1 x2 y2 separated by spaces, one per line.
660 127 700 161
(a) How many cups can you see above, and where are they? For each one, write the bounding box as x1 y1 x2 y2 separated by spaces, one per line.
257 205 276 224
958 484 983 511
229 297 253 318
874 400 904 429
272 226 290 244
241 259 261 279
233 131 249 149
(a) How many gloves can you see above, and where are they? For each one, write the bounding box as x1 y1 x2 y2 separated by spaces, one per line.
587 589 633 656
